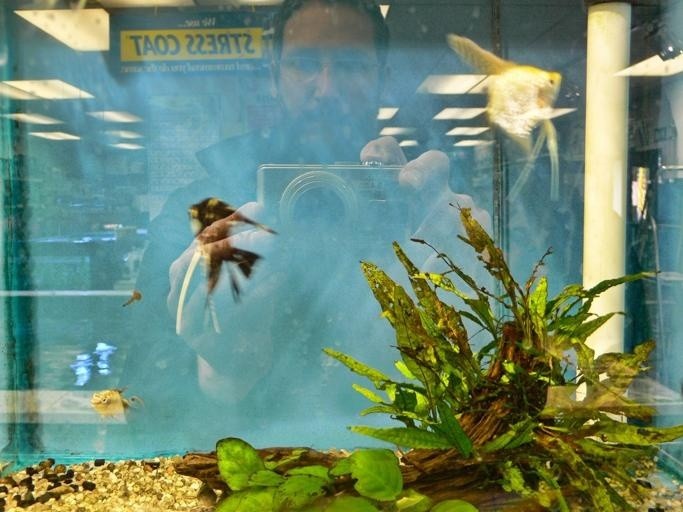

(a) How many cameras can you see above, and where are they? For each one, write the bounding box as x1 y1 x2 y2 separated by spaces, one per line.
257 160 409 247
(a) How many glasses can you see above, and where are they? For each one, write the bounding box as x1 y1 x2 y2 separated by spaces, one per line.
280 58 379 74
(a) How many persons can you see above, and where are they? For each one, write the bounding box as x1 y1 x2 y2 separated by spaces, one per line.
116 0 495 425
